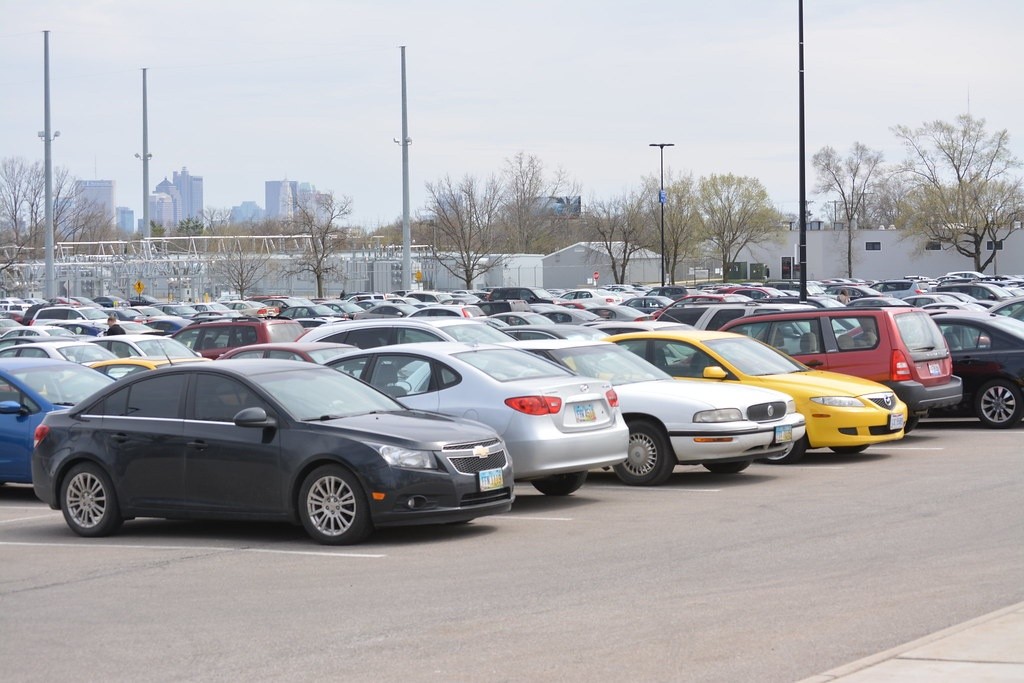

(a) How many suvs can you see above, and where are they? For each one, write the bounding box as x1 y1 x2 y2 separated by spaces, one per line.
680 305 963 433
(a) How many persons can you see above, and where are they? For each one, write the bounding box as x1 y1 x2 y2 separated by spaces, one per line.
106 316 126 335
836 289 850 304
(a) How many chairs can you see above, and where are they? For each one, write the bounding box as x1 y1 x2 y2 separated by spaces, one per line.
774 328 788 355
204 337 217 349
796 332 819 354
656 348 679 376
687 352 710 377
197 377 232 417
378 364 408 397
944 332 962 350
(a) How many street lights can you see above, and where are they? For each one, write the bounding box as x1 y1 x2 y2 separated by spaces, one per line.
134 67 151 240
38 30 60 303
828 200 842 221
393 46 413 291
649 144 674 288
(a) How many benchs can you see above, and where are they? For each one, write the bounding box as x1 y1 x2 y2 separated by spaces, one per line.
838 331 877 349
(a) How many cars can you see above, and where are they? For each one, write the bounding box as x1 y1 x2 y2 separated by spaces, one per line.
394 339 806 486
852 308 1024 430
31 358 515 546
323 341 629 494
562 330 908 464
0 358 119 488
0 272 1023 408
